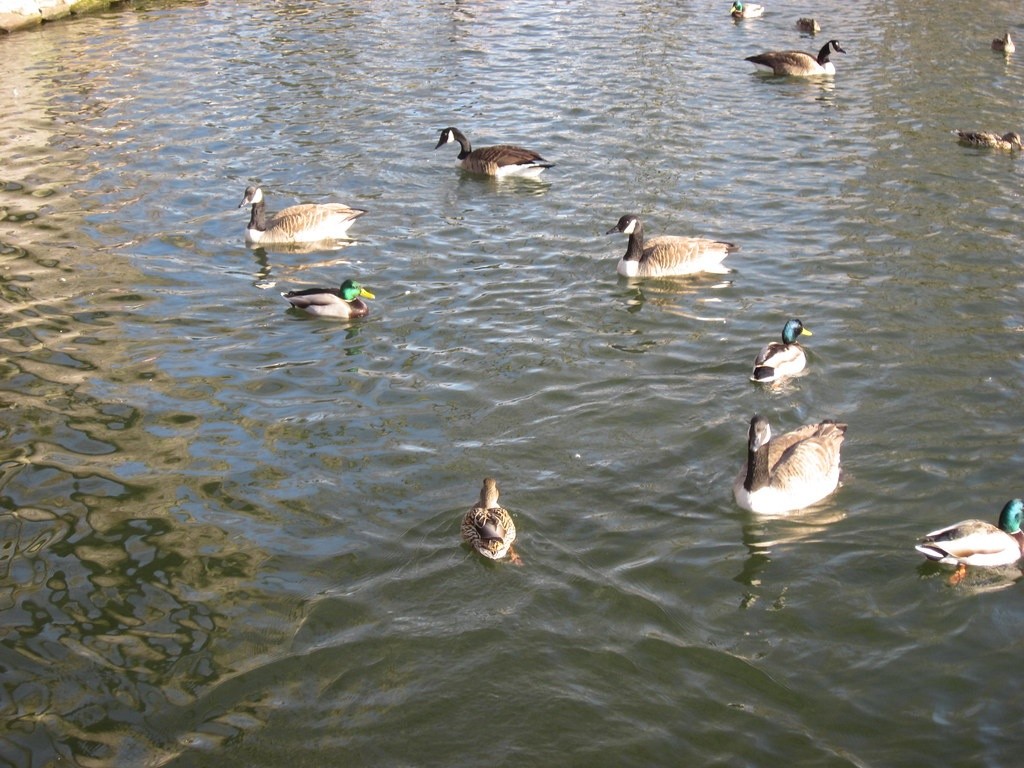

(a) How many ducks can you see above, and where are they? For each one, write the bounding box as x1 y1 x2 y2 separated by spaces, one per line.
797 17 821 35
748 316 812 388
951 128 1024 154
733 413 848 517
729 0 765 19
991 33 1016 53
435 126 557 179
606 215 741 278
914 499 1024 590
238 185 369 244
281 279 376 321
461 476 525 567
743 38 846 78
